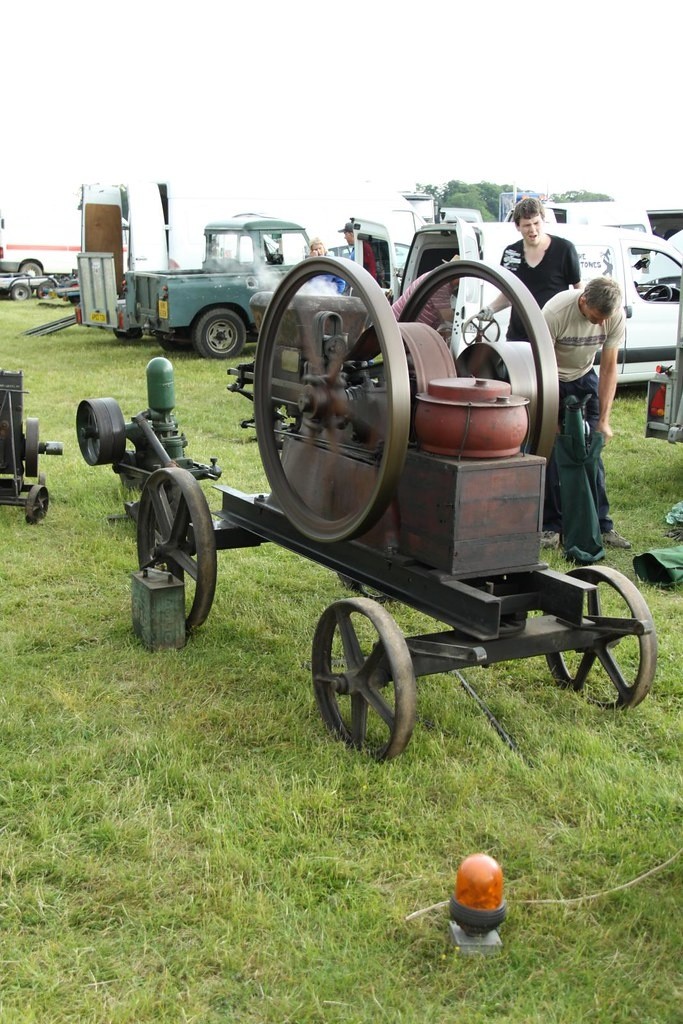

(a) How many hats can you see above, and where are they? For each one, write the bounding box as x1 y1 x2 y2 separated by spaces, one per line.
441 254 460 264
338 223 353 232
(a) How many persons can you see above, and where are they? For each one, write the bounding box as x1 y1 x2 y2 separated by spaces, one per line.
478 198 583 341
337 223 377 281
305 237 345 294
541 278 631 549
371 255 460 325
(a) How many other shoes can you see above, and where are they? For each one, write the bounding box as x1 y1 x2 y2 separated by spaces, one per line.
602 530 631 548
541 531 559 547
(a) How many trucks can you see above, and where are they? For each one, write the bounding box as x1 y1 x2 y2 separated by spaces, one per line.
400 190 446 225
77 214 313 358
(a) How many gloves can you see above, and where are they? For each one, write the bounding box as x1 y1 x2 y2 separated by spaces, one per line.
477 306 494 321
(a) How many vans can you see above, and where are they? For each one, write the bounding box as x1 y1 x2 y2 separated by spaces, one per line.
352 216 683 383
506 202 683 274
0 206 151 278
